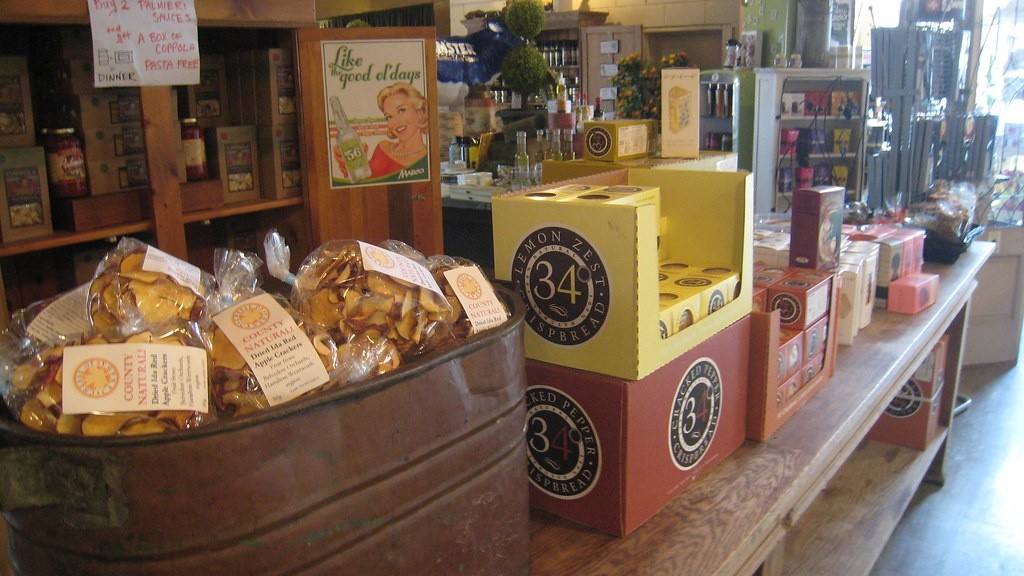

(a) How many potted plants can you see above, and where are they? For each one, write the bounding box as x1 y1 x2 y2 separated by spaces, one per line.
459 9 487 36
495 1 558 125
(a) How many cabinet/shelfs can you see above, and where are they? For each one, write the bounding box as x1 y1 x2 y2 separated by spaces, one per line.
0 0 443 323
461 10 643 113
752 67 871 214
700 70 755 172
0 241 996 576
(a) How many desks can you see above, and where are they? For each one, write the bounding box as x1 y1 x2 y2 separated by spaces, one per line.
439 194 491 212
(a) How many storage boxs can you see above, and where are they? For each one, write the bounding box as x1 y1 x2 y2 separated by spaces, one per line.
492 68 939 539
2 244 110 312
864 334 949 452
741 31 763 66
828 46 863 70
1 24 302 243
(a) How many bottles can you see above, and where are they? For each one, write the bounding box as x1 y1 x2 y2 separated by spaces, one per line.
449 41 605 191
702 83 731 151
